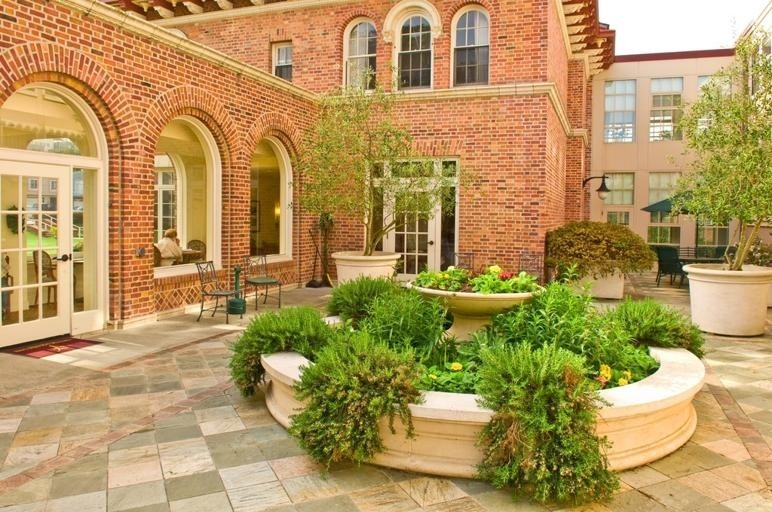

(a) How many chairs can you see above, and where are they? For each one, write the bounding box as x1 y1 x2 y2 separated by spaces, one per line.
151 240 206 269
195 261 243 324
33 249 59 311
240 246 283 310
650 245 686 287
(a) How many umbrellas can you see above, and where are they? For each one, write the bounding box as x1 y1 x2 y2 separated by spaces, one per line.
639 183 707 258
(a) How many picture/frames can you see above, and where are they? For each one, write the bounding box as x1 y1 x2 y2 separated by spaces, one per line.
250 200 260 232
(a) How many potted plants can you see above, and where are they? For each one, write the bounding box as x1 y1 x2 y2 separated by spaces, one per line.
288 57 486 301
544 217 656 301
664 20 771 338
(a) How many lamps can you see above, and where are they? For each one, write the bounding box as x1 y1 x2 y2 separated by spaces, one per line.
583 174 611 200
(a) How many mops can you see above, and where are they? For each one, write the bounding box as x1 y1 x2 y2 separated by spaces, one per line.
306 246 322 288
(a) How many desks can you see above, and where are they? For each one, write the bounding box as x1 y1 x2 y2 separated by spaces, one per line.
677 256 724 287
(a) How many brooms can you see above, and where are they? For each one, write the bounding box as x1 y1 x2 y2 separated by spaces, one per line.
309 229 335 288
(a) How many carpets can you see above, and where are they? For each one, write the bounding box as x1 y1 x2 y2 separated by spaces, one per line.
0 333 103 359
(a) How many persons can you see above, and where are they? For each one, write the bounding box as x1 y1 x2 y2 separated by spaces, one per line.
157 229 184 266
